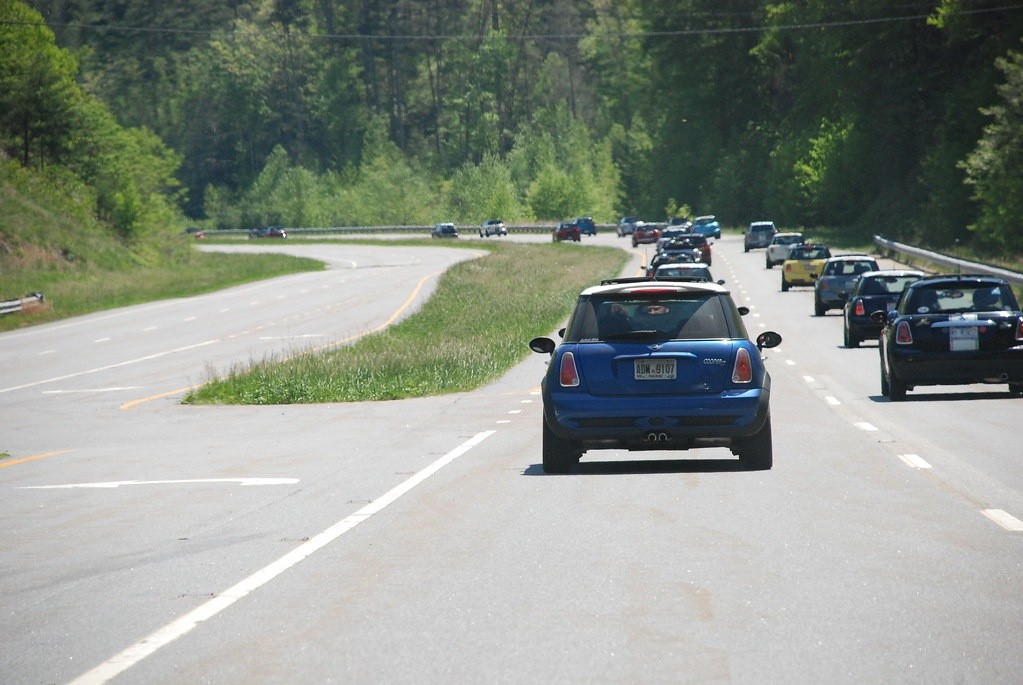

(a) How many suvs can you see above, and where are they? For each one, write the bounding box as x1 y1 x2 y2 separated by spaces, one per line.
528 280 784 474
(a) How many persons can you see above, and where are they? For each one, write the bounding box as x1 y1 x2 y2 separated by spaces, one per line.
607 304 628 321
648 306 669 314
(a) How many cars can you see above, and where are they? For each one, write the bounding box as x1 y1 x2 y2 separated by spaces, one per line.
870 273 1023 402
745 221 780 253
431 223 459 238
196 232 205 240
814 254 880 315
552 222 581 243
842 271 924 347
571 217 597 237
767 232 806 269
615 213 725 286
249 226 267 238
781 243 833 292
479 220 507 237
265 226 288 239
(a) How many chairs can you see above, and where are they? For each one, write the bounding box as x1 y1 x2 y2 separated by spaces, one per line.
854 263 869 275
971 289 996 311
